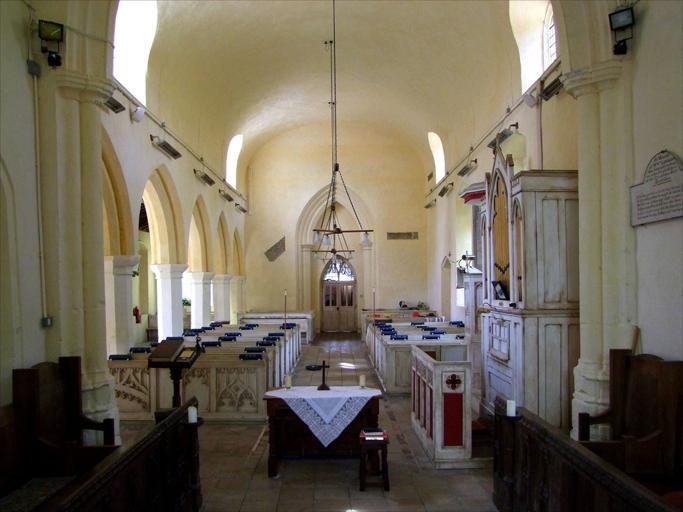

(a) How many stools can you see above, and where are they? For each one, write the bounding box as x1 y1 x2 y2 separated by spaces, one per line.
360 433 390 491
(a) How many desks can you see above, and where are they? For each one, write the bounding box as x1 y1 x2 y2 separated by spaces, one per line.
262 386 383 480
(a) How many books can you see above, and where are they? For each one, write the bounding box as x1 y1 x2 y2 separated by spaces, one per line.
359 428 388 441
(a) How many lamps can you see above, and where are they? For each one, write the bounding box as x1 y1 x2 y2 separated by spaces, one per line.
311 0 373 274
